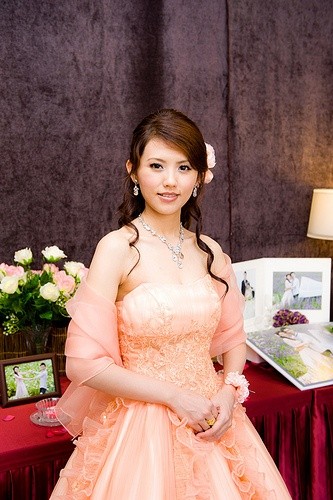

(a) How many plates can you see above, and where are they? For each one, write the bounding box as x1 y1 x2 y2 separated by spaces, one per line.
30 412 71 426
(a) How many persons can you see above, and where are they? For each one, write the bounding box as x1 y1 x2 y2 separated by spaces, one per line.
49 109 293 500
12 362 48 399
275 327 333 385
282 272 299 308
241 271 255 317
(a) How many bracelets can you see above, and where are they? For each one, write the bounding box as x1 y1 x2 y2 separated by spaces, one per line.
224 371 250 404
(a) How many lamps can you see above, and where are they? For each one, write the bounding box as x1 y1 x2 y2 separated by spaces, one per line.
305 188 333 240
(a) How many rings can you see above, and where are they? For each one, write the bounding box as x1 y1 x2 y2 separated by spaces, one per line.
206 417 216 426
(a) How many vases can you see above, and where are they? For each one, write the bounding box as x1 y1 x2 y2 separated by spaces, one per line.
19 324 54 358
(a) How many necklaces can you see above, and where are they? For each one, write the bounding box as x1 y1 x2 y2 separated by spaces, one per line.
138 212 185 269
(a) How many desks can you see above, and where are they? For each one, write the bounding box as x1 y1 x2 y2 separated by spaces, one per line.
0 362 333 500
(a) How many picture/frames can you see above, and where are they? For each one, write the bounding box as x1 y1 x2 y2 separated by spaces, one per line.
0 352 62 408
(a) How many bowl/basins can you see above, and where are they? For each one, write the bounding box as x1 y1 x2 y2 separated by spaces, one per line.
35 398 64 422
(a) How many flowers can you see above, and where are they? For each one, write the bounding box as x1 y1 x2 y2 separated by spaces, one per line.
204 143 217 185
0 242 89 336
275 308 310 329
